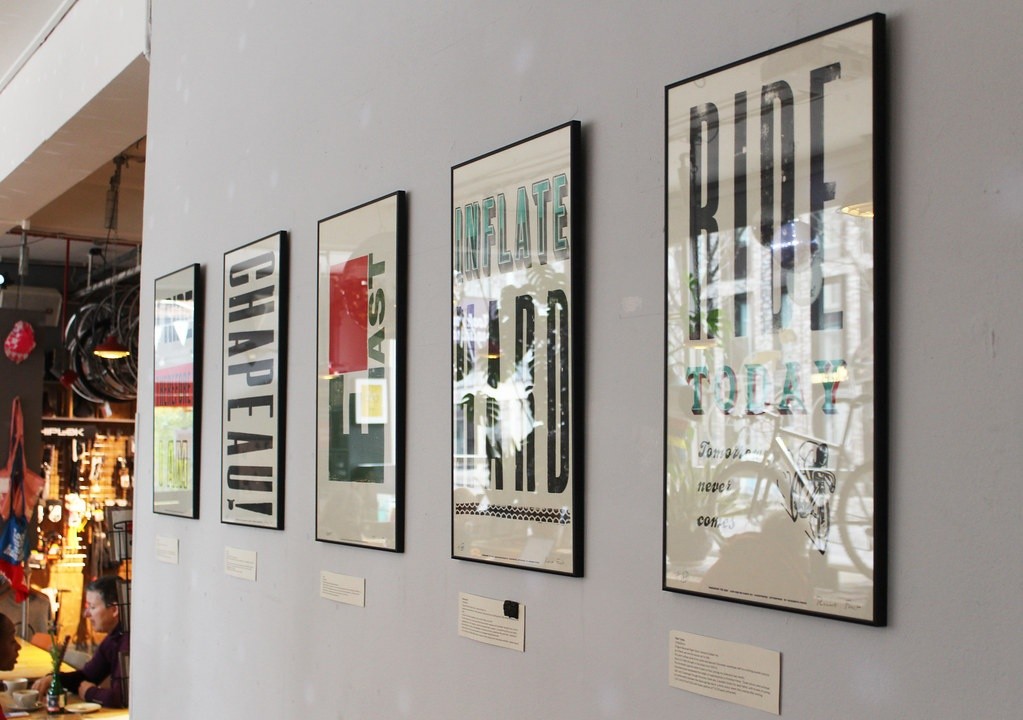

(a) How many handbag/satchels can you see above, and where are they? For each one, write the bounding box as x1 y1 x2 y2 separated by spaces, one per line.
1 397 46 523
0 440 32 567
0 558 30 604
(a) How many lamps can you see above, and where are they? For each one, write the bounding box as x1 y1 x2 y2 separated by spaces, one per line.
93 233 130 359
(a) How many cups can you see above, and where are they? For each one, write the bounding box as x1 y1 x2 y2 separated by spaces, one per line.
46 688 67 708
3 678 28 699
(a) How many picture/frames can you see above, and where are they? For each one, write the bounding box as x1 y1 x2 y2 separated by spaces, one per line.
450 118 584 578
151 262 201 520
662 10 893 630
219 229 288 531
315 190 405 554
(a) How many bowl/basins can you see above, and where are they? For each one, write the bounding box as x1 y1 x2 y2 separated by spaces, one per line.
13 690 39 708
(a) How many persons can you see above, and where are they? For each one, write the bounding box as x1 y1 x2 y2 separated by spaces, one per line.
32 575 129 709
0 612 21 720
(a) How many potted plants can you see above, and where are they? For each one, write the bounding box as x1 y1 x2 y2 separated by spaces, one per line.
46 629 70 715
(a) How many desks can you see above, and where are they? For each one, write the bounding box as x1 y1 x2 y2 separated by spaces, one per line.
1 635 128 720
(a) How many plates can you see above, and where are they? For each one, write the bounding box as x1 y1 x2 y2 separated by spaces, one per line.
64 703 102 713
6 700 44 711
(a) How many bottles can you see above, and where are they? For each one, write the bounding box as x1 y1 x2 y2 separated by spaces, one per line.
47 673 61 714
